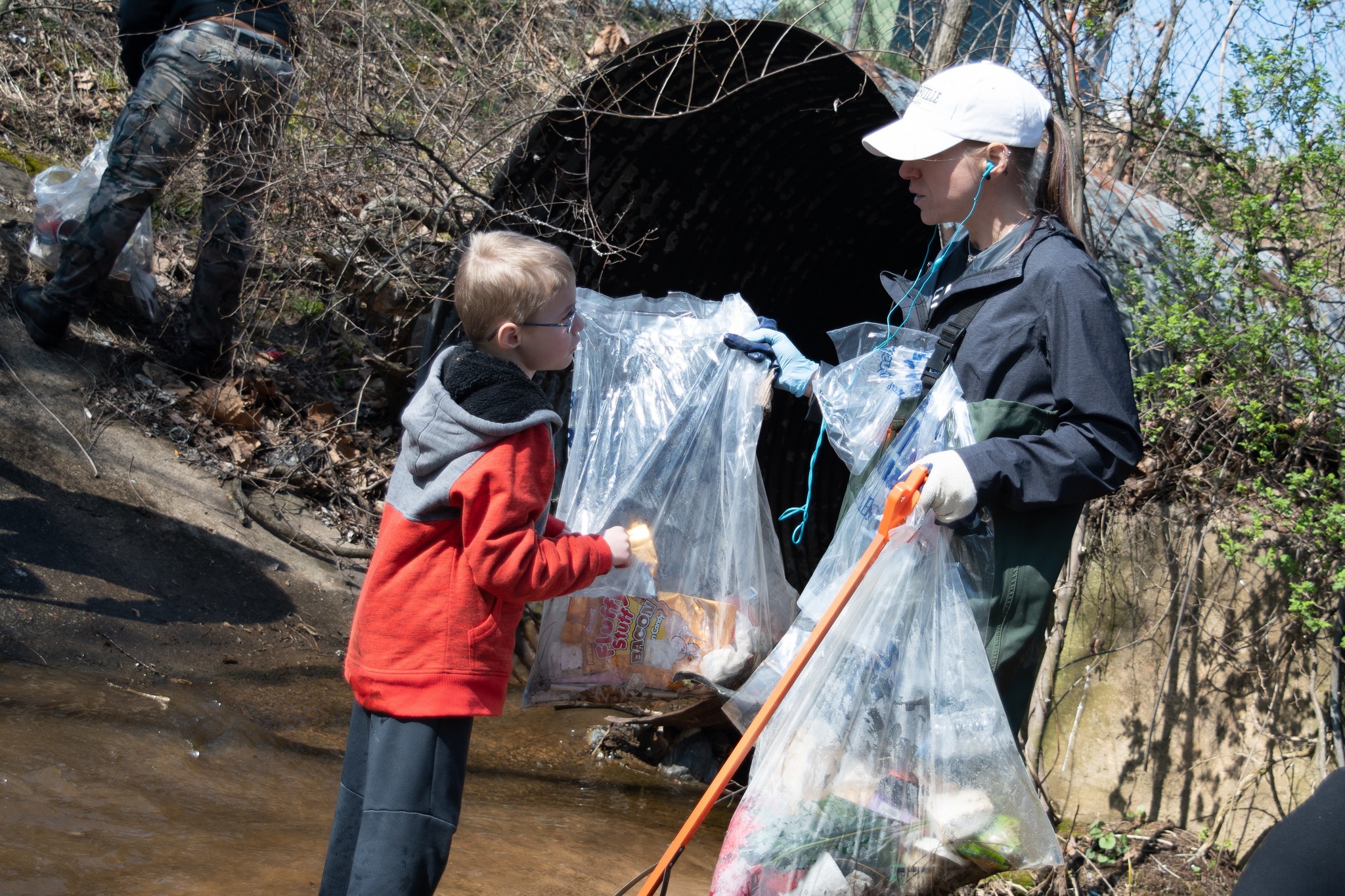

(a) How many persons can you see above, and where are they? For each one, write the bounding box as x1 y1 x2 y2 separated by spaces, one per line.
743 59 1145 896
318 231 632 896
9 0 302 383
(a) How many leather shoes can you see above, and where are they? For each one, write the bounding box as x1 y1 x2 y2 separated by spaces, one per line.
11 282 70 347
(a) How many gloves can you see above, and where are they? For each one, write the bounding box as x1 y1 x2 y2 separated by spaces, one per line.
745 328 819 398
898 450 978 523
723 317 780 376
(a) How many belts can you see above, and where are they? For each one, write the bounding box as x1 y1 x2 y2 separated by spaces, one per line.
164 18 295 64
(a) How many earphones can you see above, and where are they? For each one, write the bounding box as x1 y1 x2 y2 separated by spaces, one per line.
982 161 994 177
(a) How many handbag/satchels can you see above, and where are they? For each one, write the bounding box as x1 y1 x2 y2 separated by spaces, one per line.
703 323 1065 896
23 132 156 274
521 285 802 708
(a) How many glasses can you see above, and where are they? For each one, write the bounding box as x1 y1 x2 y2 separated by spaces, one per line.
485 301 577 343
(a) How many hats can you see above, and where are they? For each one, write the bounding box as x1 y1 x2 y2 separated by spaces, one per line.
861 59 1051 161
